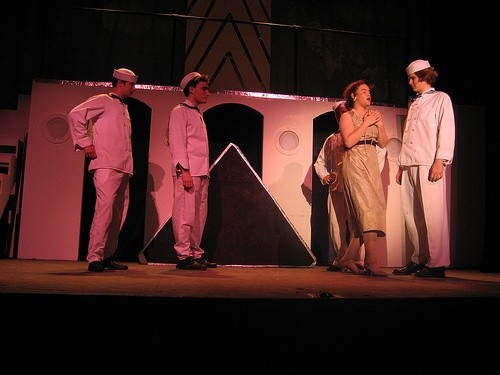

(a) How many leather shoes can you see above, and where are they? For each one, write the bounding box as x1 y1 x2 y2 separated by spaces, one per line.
392 262 426 275
192 257 217 269
102 255 129 270
175 258 206 270
414 266 445 279
88 261 104 272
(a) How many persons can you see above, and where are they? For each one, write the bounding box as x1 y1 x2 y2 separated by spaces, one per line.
69 68 138 272
339 80 389 278
169 72 218 270
314 101 387 272
393 60 455 278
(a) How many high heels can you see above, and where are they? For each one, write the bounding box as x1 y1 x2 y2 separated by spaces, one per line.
341 259 366 275
364 265 389 277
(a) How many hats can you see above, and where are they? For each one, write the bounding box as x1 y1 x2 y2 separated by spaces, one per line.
114 68 139 84
332 101 348 116
179 72 202 90
406 59 430 75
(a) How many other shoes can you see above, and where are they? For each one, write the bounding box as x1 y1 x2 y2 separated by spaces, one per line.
329 264 340 272
